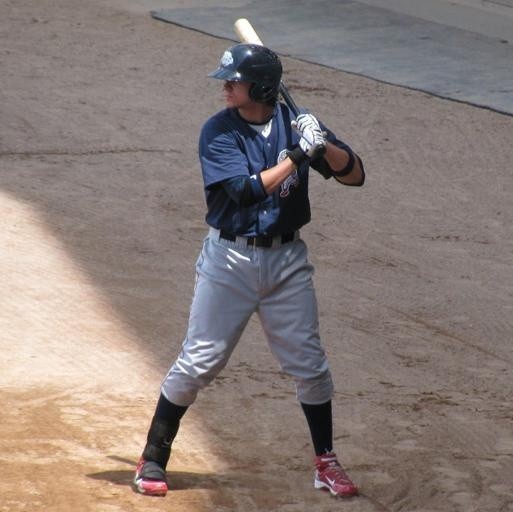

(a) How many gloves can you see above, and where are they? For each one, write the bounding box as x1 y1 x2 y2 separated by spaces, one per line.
288 113 327 168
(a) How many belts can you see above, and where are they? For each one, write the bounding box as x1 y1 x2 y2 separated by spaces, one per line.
219 227 294 247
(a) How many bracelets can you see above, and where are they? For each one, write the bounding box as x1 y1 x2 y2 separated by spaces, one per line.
288 146 308 167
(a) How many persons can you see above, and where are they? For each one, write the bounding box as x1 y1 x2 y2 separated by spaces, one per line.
133 44 365 498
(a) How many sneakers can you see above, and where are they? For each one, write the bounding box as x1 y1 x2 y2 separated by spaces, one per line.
314 452 359 498
133 456 169 496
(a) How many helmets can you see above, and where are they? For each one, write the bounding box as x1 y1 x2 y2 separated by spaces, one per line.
207 44 283 104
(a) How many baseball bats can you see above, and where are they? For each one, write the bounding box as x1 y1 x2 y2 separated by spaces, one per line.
232 17 328 159
